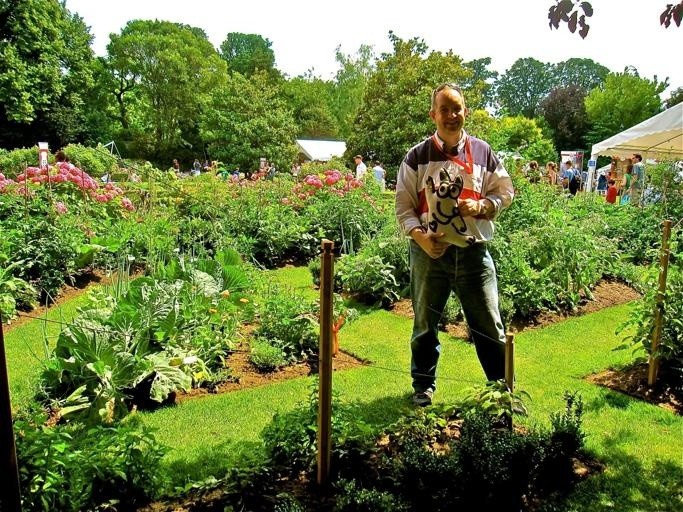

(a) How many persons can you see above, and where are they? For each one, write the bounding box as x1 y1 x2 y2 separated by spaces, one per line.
396 83 516 411
373 159 386 190
353 154 368 183
171 159 299 181
526 152 645 208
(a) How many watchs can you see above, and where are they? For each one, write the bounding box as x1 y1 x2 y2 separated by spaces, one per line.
479 200 488 216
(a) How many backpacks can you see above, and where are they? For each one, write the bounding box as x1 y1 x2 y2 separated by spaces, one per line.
569 168 581 190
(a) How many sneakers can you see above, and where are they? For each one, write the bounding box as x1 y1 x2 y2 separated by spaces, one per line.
407 387 433 407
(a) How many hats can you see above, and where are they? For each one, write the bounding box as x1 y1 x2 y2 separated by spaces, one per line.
353 155 362 160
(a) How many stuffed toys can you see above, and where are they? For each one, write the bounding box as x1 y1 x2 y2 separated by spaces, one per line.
419 167 476 248
